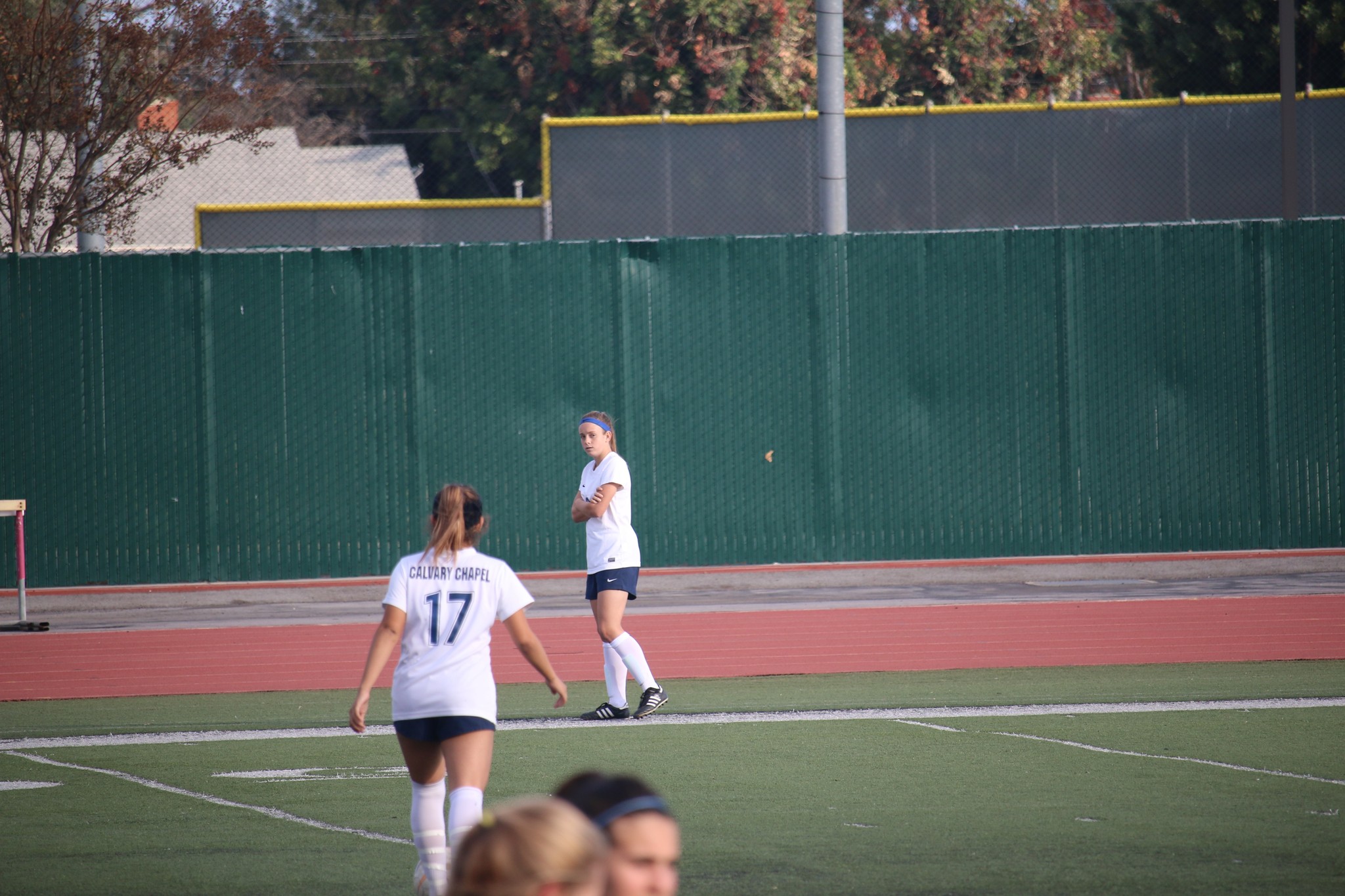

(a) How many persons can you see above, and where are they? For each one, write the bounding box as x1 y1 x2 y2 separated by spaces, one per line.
557 769 682 896
569 411 669 719
348 484 568 896
445 793 613 896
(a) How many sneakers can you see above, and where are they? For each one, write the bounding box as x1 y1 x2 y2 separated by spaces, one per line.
580 703 629 721
632 685 668 720
